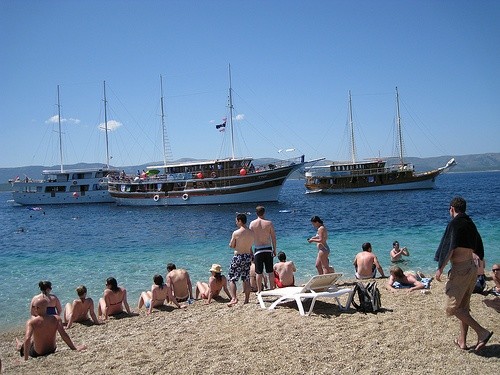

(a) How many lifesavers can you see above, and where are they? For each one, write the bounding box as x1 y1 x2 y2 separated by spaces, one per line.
211 172 217 178
73 180 77 184
154 195 159 201
182 194 188 201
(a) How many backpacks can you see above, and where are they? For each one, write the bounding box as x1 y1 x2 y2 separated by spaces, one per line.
351 281 393 315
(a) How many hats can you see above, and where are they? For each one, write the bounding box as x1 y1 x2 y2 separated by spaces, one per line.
210 263 223 273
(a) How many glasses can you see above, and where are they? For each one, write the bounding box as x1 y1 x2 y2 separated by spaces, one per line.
491 268 500 272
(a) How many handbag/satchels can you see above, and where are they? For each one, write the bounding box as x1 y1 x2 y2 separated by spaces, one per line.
474 274 493 295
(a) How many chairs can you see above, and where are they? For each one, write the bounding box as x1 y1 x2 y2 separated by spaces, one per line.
257 272 355 316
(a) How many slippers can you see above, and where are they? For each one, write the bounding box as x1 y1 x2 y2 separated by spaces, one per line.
470 330 494 352
453 338 468 350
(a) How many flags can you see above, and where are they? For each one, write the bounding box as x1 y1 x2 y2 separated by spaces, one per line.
216 121 226 132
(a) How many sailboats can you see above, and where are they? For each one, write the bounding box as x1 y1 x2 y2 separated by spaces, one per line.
7 79 123 206
302 84 459 197
96 62 327 207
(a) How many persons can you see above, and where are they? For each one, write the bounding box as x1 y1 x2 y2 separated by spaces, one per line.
164 263 194 304
30 281 62 319
228 214 254 303
97 277 131 321
434 196 493 350
136 169 146 177
490 264 500 293
478 258 485 277
308 216 330 275
16 176 20 180
249 206 276 293
63 285 102 329
196 264 232 305
353 242 384 280
390 241 409 261
26 176 28 183
387 265 424 293
242 255 267 293
272 251 296 289
138 274 172 315
16 299 85 361
